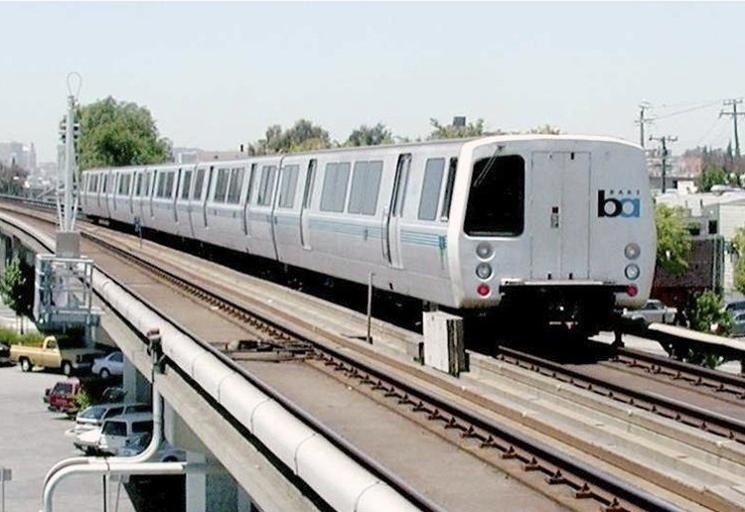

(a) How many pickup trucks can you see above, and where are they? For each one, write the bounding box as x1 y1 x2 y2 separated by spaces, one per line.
9 334 106 376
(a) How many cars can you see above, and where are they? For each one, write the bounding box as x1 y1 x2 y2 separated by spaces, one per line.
716 299 745 334
45 349 188 462
626 298 677 324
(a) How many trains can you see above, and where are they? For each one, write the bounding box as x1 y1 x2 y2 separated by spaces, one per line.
80 132 657 353
